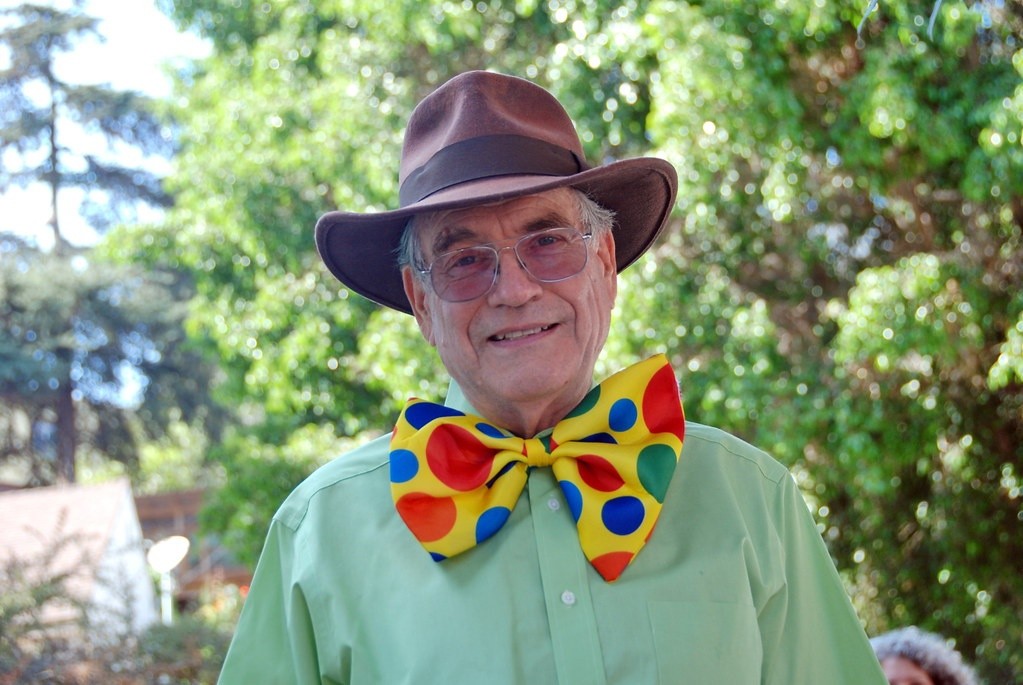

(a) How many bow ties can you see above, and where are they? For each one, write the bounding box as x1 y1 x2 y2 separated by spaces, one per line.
386 352 683 582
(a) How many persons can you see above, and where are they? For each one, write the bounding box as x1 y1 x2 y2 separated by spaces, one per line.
866 626 977 685
215 71 889 684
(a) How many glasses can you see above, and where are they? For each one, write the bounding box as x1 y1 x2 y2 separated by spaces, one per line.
413 228 594 303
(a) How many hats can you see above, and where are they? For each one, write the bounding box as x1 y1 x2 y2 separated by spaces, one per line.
314 69 677 316
